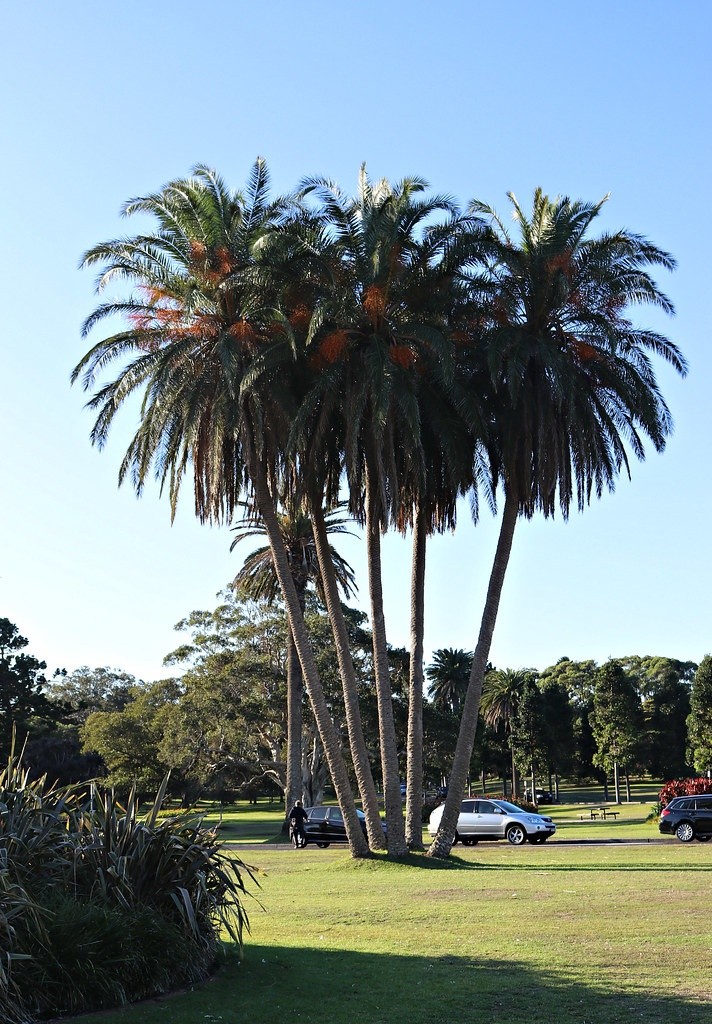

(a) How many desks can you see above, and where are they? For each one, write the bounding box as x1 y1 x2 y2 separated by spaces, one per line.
589 807 610 820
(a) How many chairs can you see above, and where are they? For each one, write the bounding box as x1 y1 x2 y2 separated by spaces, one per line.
481 805 491 813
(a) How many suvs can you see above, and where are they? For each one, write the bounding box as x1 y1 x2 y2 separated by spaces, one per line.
426 798 557 846
658 794 712 842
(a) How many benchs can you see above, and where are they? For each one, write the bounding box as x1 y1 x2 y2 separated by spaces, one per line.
577 813 599 820
593 812 619 820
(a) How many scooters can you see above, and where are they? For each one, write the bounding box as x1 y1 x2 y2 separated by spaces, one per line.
289 820 311 851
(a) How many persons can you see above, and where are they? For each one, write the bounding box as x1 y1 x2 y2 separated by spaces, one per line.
287 799 308 849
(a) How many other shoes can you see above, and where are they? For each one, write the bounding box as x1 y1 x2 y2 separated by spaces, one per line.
294 846 298 849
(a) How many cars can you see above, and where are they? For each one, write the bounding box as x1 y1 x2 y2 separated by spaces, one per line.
435 787 448 798
299 806 388 848
523 787 552 806
400 785 408 794
